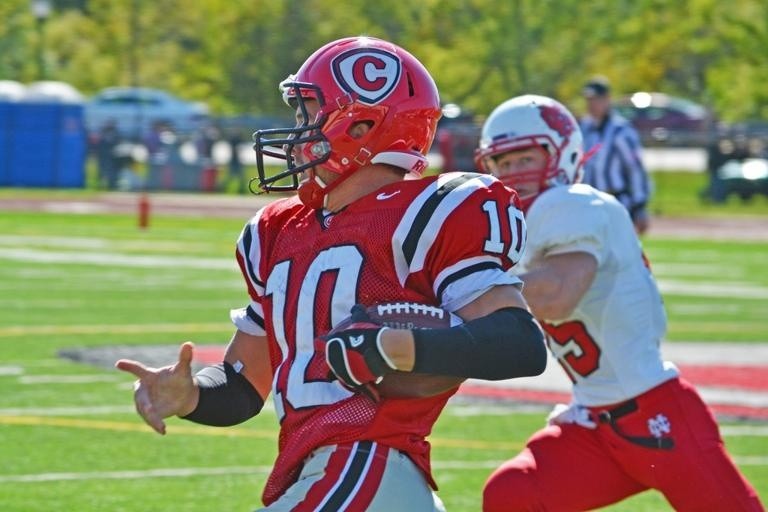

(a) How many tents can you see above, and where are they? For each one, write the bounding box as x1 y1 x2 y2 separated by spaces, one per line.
0 81 85 186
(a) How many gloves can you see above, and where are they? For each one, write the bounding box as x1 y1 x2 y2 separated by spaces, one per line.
313 304 398 403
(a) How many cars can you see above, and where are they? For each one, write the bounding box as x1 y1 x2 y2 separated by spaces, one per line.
615 92 768 202
82 88 208 145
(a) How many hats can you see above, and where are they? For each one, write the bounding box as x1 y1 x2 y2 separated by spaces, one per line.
582 77 611 97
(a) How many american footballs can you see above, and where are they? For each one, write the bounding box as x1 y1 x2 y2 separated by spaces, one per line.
329 302 471 398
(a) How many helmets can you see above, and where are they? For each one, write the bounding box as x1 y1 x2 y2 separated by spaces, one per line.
282 35 444 210
479 94 585 186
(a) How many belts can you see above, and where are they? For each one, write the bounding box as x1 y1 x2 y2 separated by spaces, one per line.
578 398 639 426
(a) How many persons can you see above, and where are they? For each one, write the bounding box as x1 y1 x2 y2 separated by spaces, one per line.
115 36 547 512
97 117 246 193
475 94 768 512
578 78 652 233
